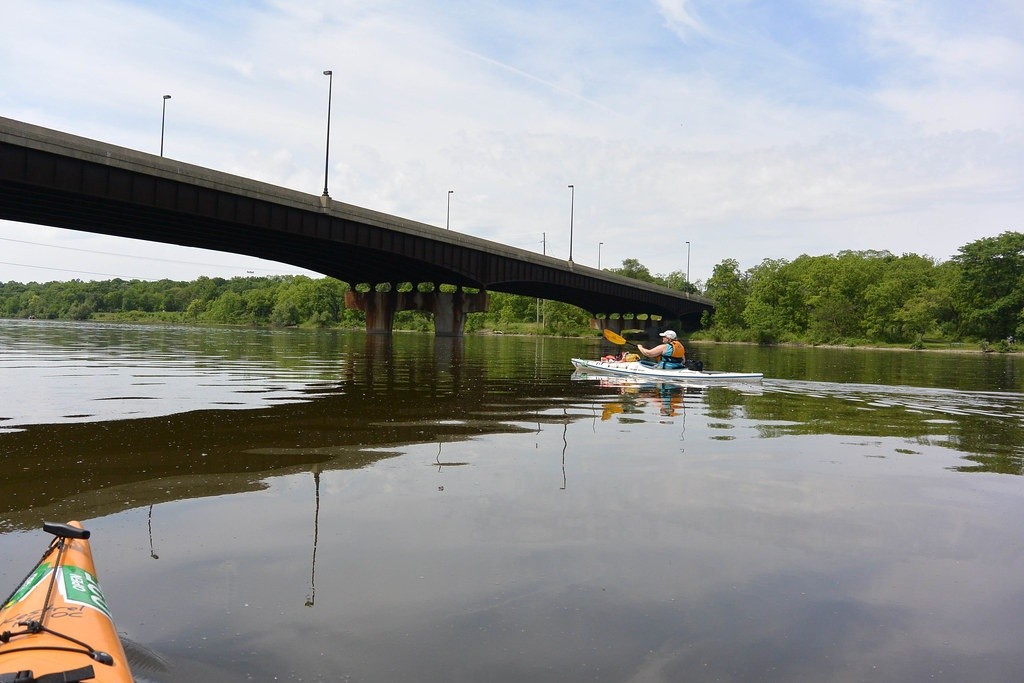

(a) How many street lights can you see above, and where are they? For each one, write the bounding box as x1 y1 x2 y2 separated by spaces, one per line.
686 241 690 283
568 184 574 263
598 242 603 270
447 191 453 230
322 70 333 196
161 94 171 157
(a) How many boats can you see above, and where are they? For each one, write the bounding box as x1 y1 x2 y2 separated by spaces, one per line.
571 357 764 383
0 519 135 683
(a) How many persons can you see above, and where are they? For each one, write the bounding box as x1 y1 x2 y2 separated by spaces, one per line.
637 330 685 369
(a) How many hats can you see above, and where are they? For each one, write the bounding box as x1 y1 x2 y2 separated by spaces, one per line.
659 330 677 340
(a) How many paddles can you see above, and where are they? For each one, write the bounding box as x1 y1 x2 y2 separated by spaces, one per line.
602 328 703 371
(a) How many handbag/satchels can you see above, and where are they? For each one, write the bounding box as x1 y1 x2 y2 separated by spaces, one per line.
687 358 703 371
